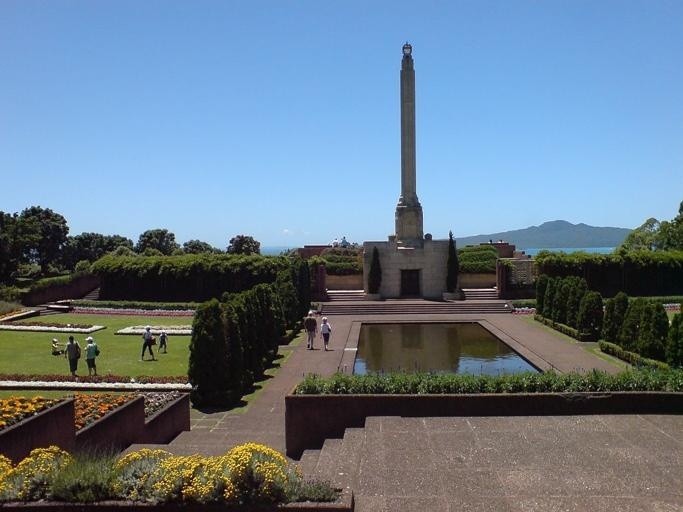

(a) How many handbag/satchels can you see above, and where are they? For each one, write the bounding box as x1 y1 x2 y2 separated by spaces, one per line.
328 324 332 332
76 342 80 358
151 336 157 345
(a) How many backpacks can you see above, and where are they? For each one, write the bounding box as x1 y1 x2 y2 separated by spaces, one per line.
95 345 100 356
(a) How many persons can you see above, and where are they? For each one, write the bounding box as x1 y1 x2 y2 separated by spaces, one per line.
139 325 154 361
51 338 64 355
156 330 168 353
339 236 348 247
331 238 338 248
318 316 331 350
303 311 318 349
82 336 97 376
63 334 81 377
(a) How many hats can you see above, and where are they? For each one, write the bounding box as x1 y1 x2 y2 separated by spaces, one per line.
309 311 313 315
85 337 94 341
145 326 150 329
